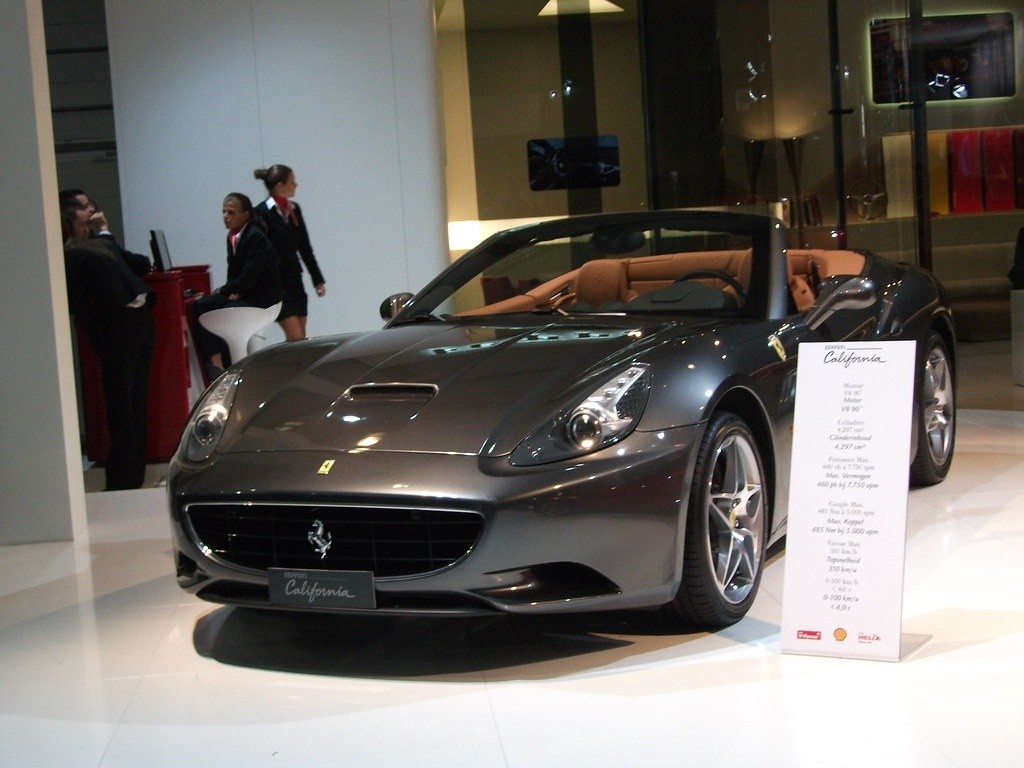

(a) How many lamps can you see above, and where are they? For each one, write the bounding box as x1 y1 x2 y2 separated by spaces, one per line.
743 141 766 213
783 137 806 250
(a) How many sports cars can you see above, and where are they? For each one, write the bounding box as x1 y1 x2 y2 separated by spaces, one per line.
166 205 959 627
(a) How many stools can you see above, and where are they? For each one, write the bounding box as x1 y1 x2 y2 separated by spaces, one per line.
199 301 282 365
248 322 287 356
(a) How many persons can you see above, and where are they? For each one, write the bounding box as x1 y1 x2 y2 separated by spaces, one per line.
60 189 158 490
252 164 326 341
192 192 285 381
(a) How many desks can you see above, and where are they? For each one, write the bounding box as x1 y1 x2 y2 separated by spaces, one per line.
74 265 211 462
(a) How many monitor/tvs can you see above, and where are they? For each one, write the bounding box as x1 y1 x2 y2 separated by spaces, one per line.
150 230 172 271
